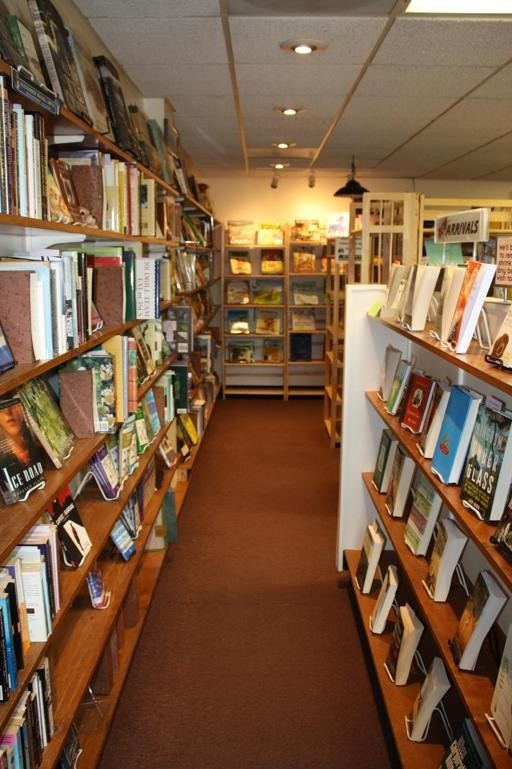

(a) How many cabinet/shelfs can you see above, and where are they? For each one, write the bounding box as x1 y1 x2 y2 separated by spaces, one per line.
218 222 327 396
420 191 512 303
325 190 421 456
0 56 215 769
333 283 512 767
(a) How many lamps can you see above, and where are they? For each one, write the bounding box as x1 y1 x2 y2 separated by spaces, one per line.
307 168 316 188
334 150 370 201
270 172 280 190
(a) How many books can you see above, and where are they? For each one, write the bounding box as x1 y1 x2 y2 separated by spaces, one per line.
354 519 490 768
381 260 511 369
0 0 219 250
0 235 228 507
229 213 350 362
0 328 216 769
371 428 512 749
377 343 512 560
